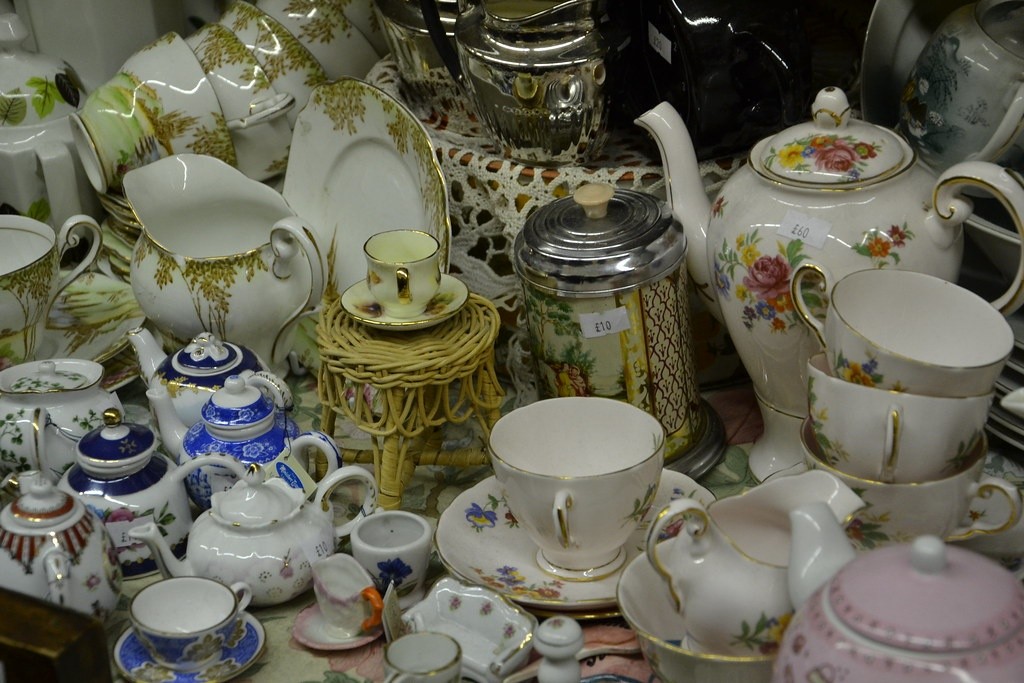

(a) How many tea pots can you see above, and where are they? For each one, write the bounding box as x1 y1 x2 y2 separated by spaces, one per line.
633 86 1024 480
0 321 1023 683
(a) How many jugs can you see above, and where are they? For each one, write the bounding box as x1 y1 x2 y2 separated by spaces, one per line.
640 461 862 652
114 153 324 377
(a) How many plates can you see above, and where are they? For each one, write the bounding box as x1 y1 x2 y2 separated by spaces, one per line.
335 281 472 327
289 608 392 654
109 613 269 682
30 268 145 360
281 77 450 299
435 472 717 610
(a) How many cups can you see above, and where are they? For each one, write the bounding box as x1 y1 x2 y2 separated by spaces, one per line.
788 262 1021 548
0 0 247 669
615 539 782 682
362 228 443 316
350 506 435 609
492 394 672 578
